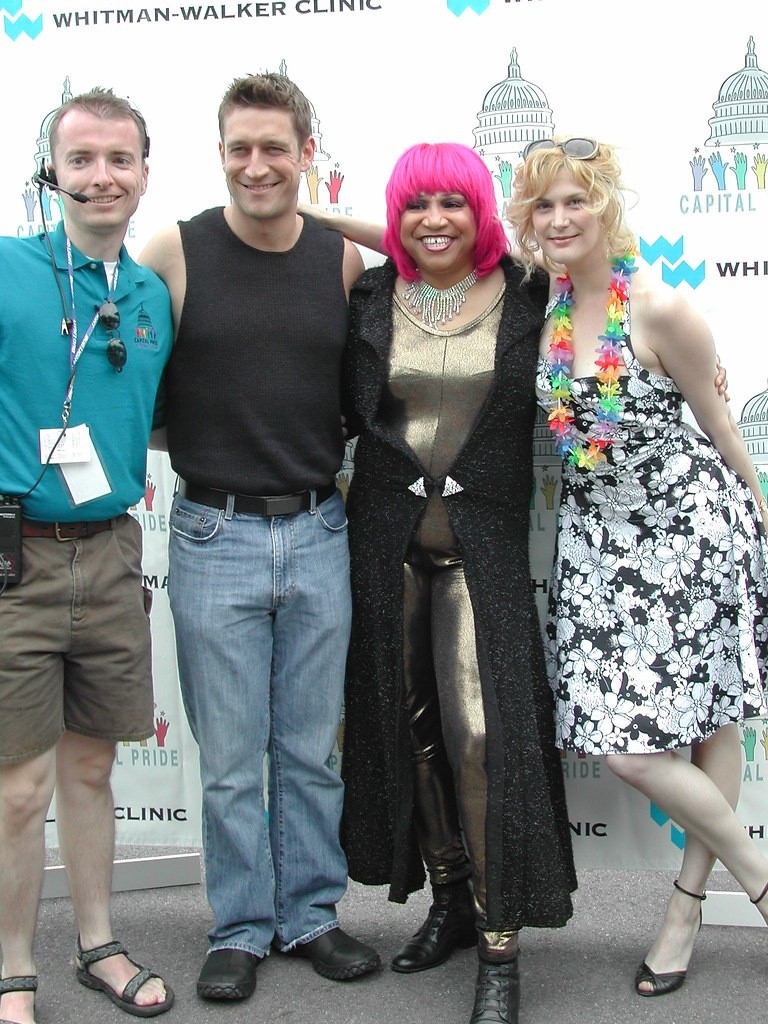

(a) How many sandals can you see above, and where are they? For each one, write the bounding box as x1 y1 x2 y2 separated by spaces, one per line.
0 976 37 1024
74 935 174 1017
637 880 706 996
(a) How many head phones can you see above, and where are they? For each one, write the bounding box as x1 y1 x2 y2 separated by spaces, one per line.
40 99 151 190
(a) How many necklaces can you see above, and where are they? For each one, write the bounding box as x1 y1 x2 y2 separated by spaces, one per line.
401 269 477 326
545 247 641 473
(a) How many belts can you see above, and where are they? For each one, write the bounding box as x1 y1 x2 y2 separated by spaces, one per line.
21 514 128 537
179 476 336 514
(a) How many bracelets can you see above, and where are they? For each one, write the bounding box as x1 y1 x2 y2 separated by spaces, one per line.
758 496 767 512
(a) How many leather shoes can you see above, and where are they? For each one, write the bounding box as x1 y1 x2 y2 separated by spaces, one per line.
391 884 478 973
271 929 381 979
198 949 266 1000
469 963 519 1024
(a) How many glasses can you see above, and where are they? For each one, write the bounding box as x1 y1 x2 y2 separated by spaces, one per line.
100 299 127 372
522 137 598 161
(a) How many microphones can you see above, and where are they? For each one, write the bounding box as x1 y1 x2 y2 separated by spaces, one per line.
35 176 87 203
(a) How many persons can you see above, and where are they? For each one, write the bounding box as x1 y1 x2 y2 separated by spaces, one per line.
340 139 732 1024
136 70 383 1004
0 87 349 1024
294 134 768 999
